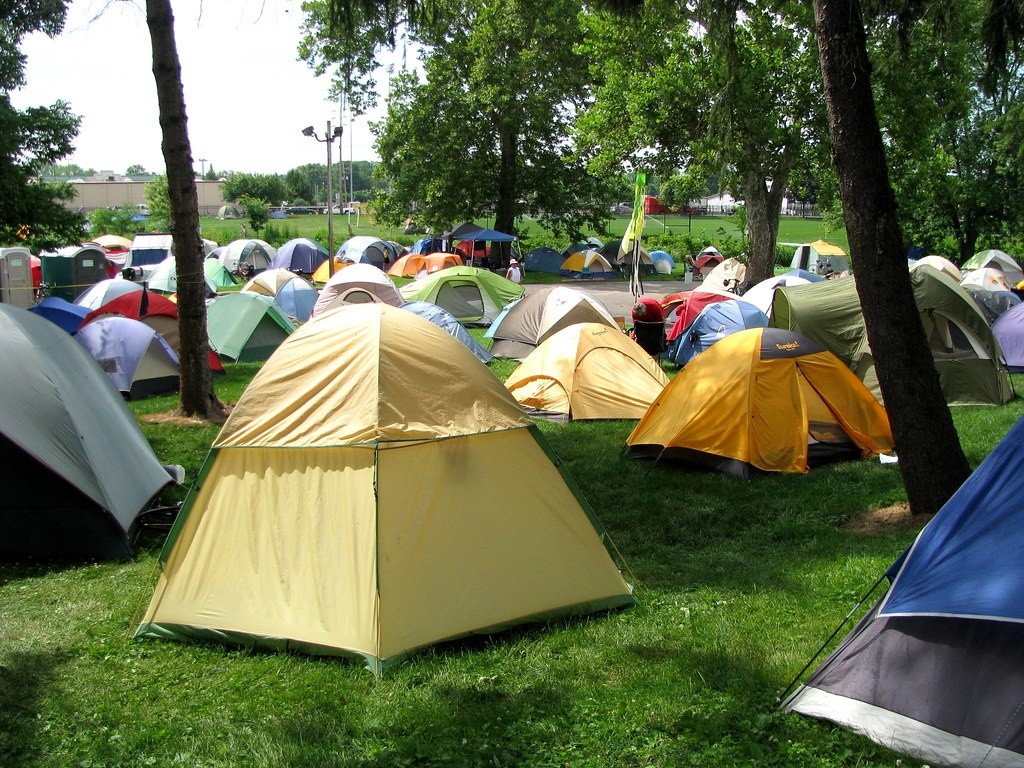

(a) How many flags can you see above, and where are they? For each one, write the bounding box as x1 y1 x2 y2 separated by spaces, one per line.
621 172 645 255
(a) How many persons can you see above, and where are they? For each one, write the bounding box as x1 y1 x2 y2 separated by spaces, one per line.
632 298 666 323
383 246 390 272
506 259 522 284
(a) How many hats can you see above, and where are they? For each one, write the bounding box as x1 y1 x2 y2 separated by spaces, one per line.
509 258 518 265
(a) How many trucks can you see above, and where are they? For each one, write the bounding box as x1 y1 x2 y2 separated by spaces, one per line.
118 204 153 217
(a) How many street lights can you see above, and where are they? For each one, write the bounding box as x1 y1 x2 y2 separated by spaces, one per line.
302 121 343 279
199 158 208 180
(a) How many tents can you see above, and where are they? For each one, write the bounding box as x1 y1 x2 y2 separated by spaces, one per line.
772 412 1024 768
0 206 1024 679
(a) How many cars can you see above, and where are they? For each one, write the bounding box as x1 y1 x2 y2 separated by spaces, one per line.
283 207 318 216
323 203 356 215
